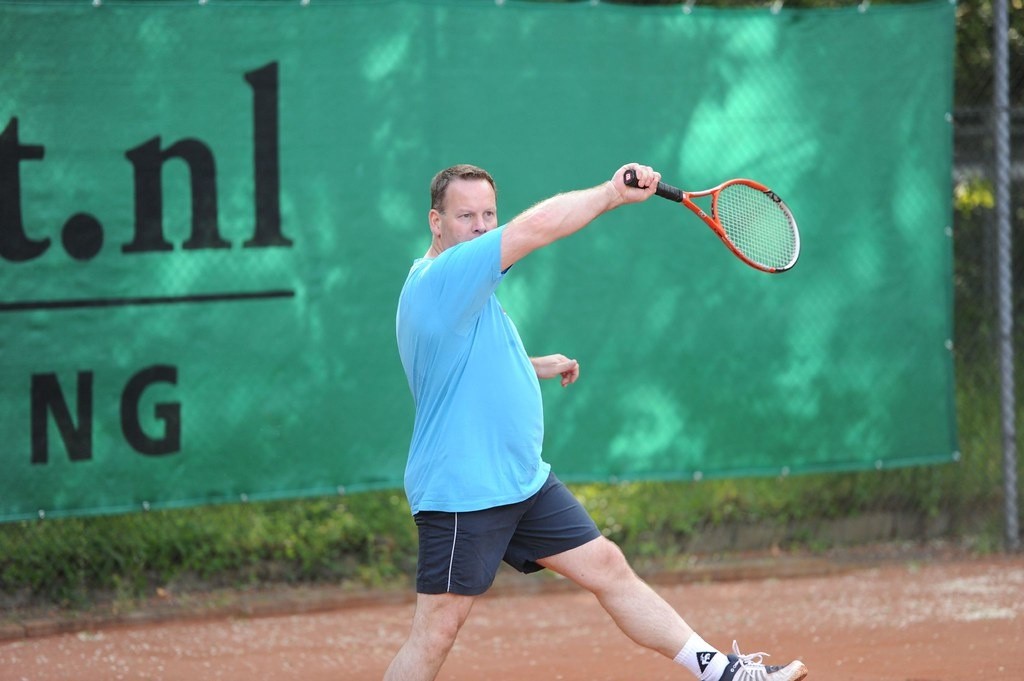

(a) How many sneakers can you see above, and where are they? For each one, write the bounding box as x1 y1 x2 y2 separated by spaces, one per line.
719 640 807 681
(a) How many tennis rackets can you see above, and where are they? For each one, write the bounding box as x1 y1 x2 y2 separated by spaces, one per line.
624 169 800 275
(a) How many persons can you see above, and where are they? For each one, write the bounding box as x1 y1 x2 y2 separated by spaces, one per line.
380 162 807 681
